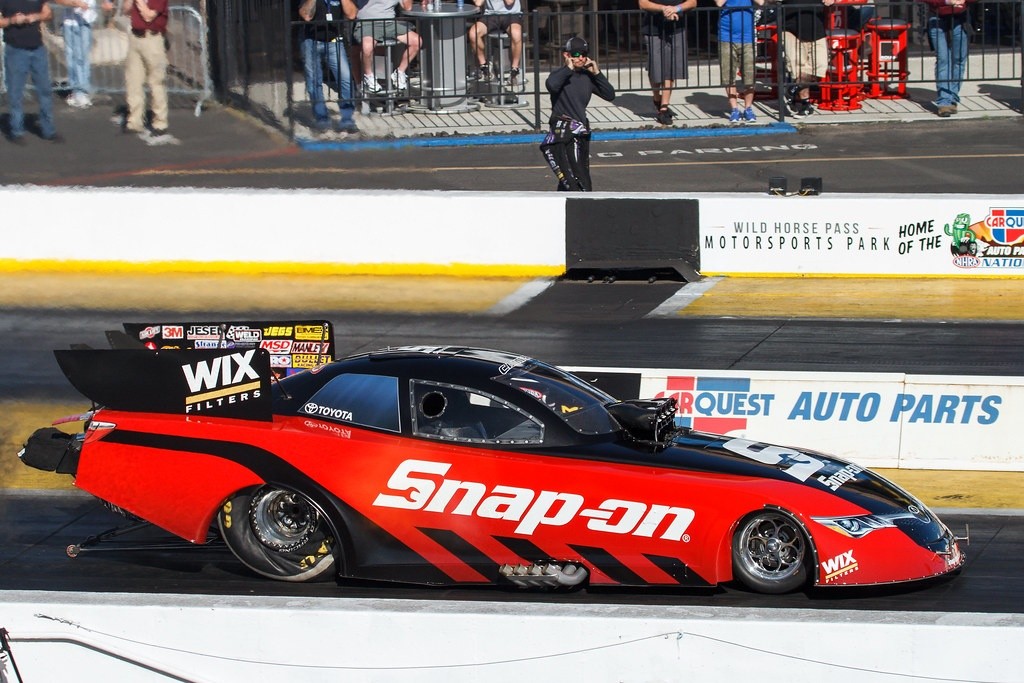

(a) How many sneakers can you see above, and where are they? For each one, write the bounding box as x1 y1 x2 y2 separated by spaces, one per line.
938 107 950 117
391 67 409 90
318 117 329 133
783 94 799 114
794 98 815 119
950 103 957 113
656 110 674 125
478 60 495 82
337 120 361 133
730 108 742 123
743 106 757 123
654 95 674 119
8 134 27 147
508 67 520 85
363 73 383 93
42 134 66 145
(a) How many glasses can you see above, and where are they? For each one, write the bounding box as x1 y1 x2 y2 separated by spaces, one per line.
567 50 587 57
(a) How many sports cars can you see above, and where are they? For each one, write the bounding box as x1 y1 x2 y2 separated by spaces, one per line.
16 317 973 595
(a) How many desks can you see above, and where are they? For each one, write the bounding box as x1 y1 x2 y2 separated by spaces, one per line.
803 0 869 105
399 2 483 116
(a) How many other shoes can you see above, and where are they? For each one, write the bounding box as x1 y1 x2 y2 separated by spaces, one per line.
150 129 168 138
122 125 143 136
66 93 93 107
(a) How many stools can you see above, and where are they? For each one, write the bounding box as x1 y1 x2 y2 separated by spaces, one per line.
866 18 911 100
737 20 781 102
818 28 863 112
484 26 531 111
356 36 410 117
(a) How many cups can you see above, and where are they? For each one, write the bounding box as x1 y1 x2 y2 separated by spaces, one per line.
421 0 443 10
457 0 464 6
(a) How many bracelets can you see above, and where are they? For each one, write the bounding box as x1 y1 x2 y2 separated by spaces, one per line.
8 17 11 26
676 3 683 13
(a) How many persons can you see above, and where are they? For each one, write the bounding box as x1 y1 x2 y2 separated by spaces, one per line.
924 0 970 116
470 0 524 81
299 0 359 132
57 0 98 109
352 0 423 93
540 37 616 192
0 0 64 145
119 0 171 137
637 0 698 124
714 0 766 122
776 0 836 119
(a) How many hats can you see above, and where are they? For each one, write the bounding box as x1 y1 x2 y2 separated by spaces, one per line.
565 38 589 52
